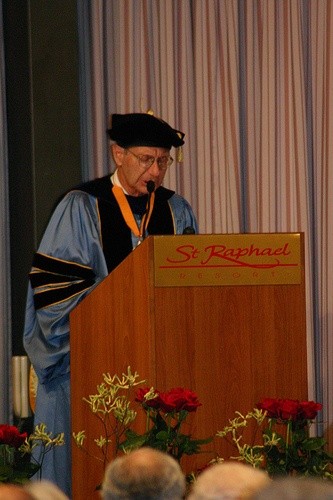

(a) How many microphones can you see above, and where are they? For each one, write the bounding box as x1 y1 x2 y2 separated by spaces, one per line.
142 179 156 240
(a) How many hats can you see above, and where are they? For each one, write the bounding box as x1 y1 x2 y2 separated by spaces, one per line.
106 112 185 149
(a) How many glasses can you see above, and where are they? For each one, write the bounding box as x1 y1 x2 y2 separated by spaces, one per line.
127 148 174 169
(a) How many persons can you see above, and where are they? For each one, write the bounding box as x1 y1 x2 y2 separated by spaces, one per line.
101 447 186 500
257 478 333 500
24 110 196 500
186 462 268 500
0 478 69 500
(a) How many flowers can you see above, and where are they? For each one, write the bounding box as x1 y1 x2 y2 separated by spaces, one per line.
73 366 215 491
0 422 66 484
216 397 333 483
